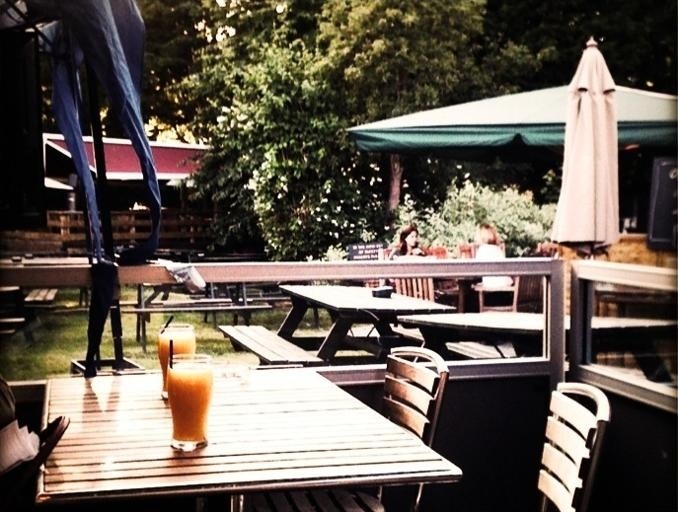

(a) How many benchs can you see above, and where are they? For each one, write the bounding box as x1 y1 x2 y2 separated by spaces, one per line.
118 296 325 364
23 287 60 305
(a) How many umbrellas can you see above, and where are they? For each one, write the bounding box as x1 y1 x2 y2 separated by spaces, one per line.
551 35 620 261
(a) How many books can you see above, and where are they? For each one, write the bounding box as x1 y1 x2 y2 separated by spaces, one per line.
0 419 40 473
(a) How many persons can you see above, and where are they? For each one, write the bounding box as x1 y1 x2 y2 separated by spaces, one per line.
388 225 427 294
465 223 513 313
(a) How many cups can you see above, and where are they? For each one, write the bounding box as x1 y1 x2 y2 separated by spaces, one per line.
158 323 214 453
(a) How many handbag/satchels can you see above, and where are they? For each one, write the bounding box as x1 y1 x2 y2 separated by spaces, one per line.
0 415 71 511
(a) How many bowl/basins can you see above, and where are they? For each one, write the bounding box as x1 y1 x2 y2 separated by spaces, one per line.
372 284 394 298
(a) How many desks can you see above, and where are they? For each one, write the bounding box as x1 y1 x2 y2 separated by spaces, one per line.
36 364 464 510
0 256 89 308
275 285 678 382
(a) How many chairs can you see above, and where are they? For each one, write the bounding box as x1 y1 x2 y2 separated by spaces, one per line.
381 240 565 312
536 382 613 512
246 344 452 512
